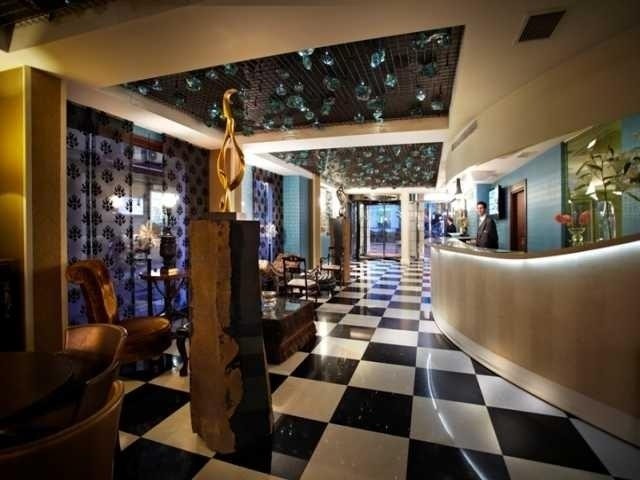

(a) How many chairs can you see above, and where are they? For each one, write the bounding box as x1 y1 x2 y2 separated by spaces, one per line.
281 253 321 303
175 319 193 376
322 246 345 290
1 381 126 480
33 324 129 424
64 259 173 382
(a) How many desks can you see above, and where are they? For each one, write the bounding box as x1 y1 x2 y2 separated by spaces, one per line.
317 277 337 302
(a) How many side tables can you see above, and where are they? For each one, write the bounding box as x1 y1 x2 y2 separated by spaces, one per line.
138 267 187 339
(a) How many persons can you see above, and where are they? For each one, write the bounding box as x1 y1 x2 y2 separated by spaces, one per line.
475 201 499 250
266 259 275 271
279 257 287 272
446 217 456 237
